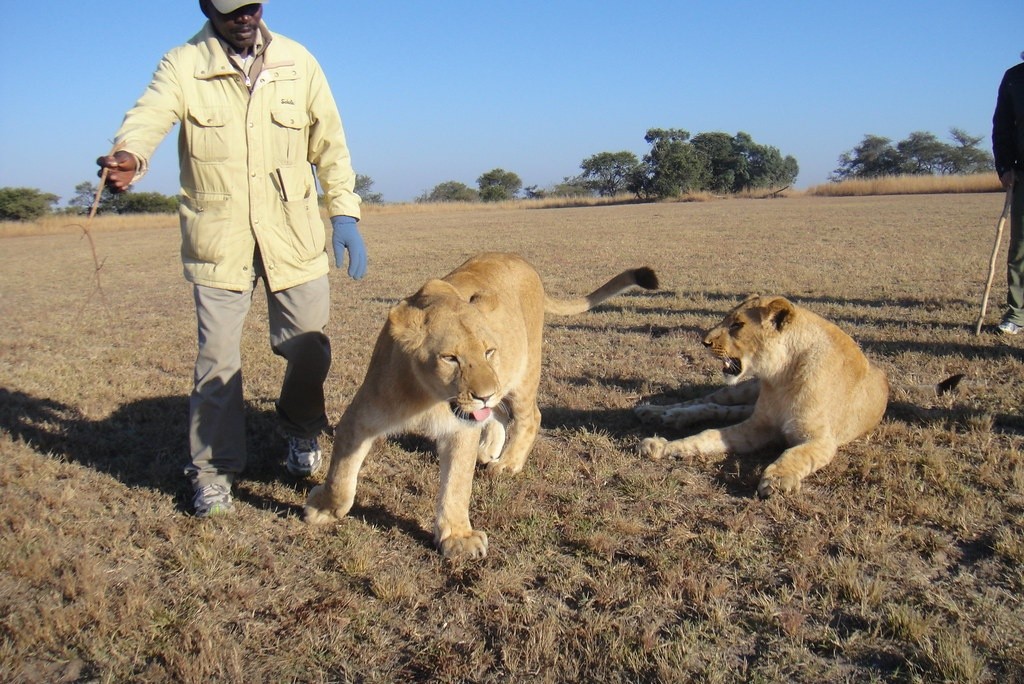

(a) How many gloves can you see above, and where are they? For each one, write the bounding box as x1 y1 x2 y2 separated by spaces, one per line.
330 215 367 281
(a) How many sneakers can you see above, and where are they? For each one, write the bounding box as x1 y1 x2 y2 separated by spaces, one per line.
996 320 1024 335
279 425 322 480
184 471 237 520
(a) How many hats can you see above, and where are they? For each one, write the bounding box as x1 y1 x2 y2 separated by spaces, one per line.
210 0 262 14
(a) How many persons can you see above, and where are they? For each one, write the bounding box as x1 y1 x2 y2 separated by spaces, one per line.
992 52 1024 336
95 1 370 518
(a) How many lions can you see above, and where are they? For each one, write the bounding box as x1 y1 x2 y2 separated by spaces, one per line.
633 294 966 502
302 252 659 558
